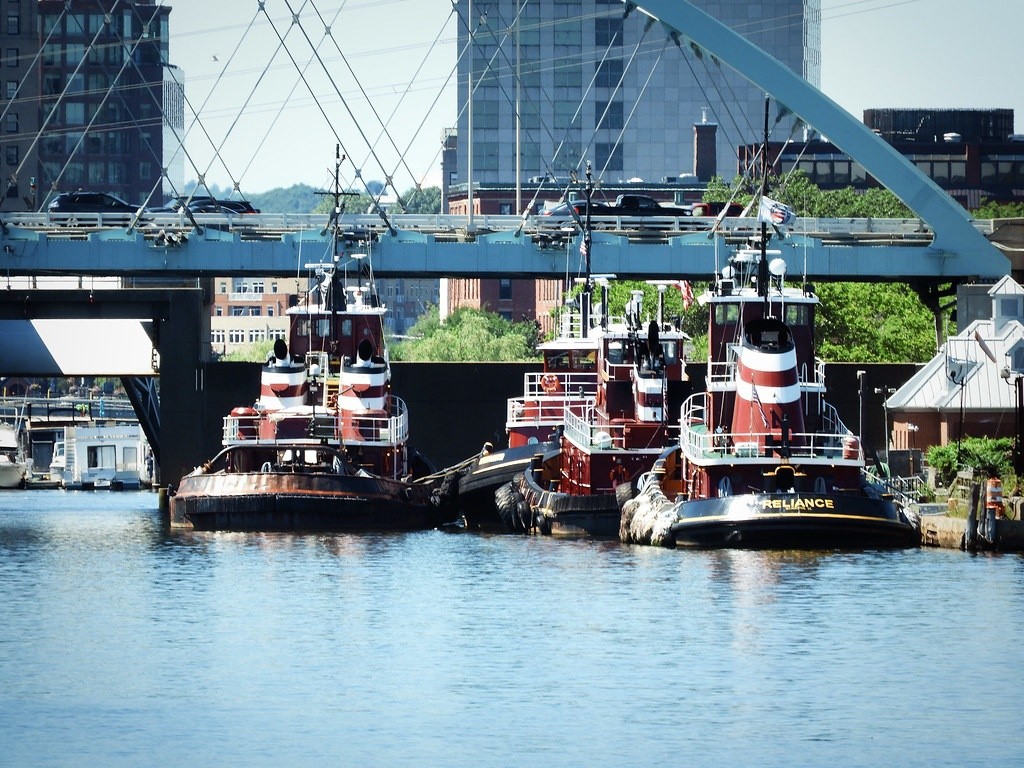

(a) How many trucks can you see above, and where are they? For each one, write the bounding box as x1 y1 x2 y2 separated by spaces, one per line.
683 201 743 230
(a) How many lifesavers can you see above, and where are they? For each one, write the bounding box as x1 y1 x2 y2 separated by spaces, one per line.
609 465 629 484
536 515 550 536
493 483 539 532
541 374 558 391
616 481 636 513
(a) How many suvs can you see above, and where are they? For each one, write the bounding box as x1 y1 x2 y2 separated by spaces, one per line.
48 191 262 235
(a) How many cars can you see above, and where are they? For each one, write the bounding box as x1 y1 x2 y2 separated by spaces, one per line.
539 200 627 215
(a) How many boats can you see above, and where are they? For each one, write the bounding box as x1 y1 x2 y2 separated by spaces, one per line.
173 93 924 550
0 386 65 488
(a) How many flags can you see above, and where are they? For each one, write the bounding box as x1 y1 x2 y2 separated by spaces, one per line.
758 196 796 227
675 281 695 310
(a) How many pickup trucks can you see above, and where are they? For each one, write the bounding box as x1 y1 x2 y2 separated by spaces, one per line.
602 195 691 216
